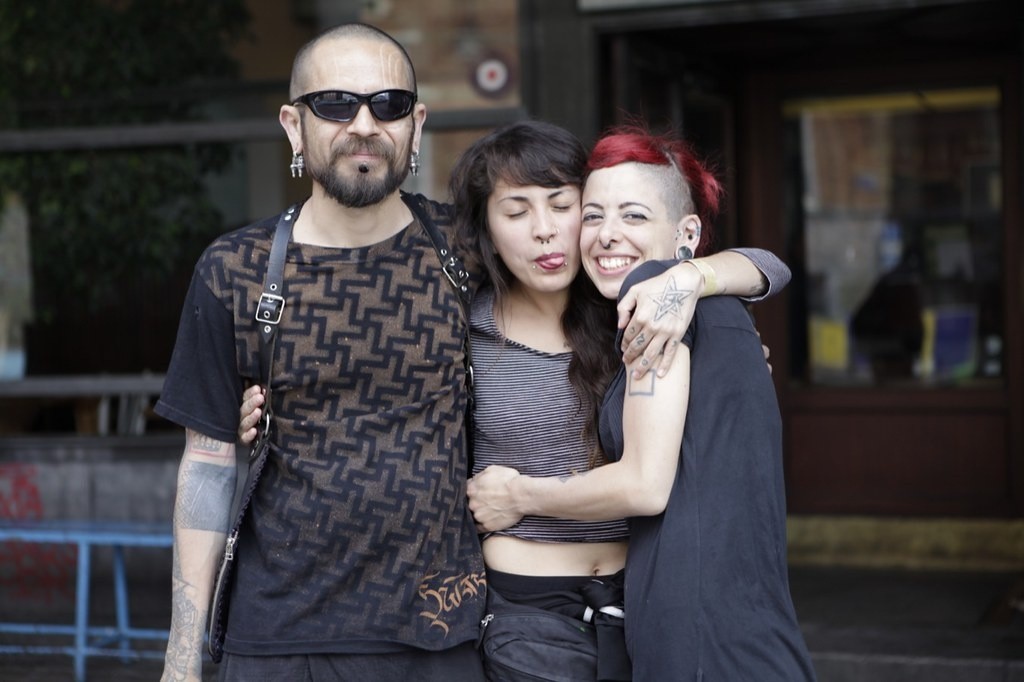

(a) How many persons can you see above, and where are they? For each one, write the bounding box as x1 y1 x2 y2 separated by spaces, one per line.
154 23 491 682
236 121 635 682
466 107 816 682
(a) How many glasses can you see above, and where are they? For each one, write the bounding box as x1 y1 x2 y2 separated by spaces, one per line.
294 89 417 122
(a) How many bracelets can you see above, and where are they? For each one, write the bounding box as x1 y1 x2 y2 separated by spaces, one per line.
678 258 715 297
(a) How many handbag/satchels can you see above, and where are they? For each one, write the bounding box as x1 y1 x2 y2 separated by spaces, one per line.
475 586 597 682
